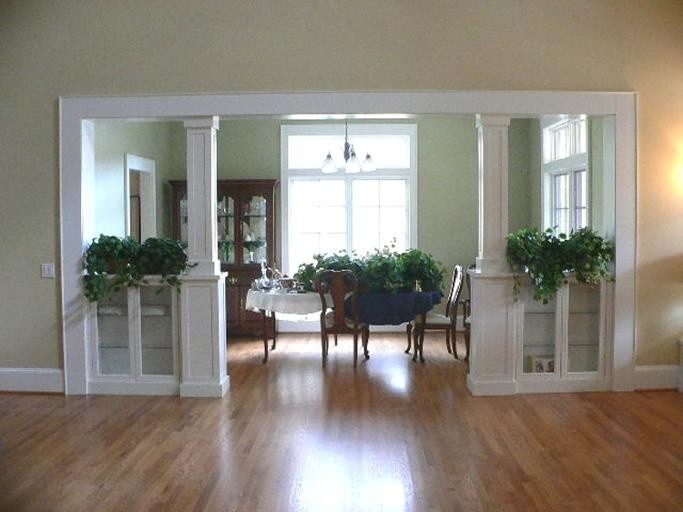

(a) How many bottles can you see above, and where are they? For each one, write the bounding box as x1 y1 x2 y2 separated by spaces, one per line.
258 258 270 290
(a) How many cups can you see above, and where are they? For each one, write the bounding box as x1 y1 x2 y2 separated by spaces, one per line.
295 283 304 291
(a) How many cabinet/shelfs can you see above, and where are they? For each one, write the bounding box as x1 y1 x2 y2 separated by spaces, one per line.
516 276 607 380
168 180 280 337
91 281 179 382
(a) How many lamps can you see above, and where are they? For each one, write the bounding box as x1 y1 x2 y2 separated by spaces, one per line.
321 122 376 173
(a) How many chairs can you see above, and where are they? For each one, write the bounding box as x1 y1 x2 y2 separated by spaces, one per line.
459 264 476 362
316 269 370 366
404 265 465 361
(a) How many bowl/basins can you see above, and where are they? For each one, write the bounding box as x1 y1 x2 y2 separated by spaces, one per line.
279 278 296 289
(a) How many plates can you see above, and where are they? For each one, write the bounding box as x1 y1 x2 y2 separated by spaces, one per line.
254 221 266 240
229 247 250 264
227 219 249 242
252 246 267 263
